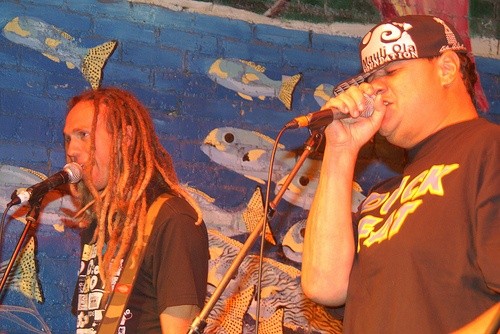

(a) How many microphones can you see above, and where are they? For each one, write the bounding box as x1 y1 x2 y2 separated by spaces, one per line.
6 162 83 205
286 93 374 128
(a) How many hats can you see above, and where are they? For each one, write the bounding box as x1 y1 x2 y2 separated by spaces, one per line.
332 15 468 97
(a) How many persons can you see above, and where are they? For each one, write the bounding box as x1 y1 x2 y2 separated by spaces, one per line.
62 84 211 334
299 13 500 334
